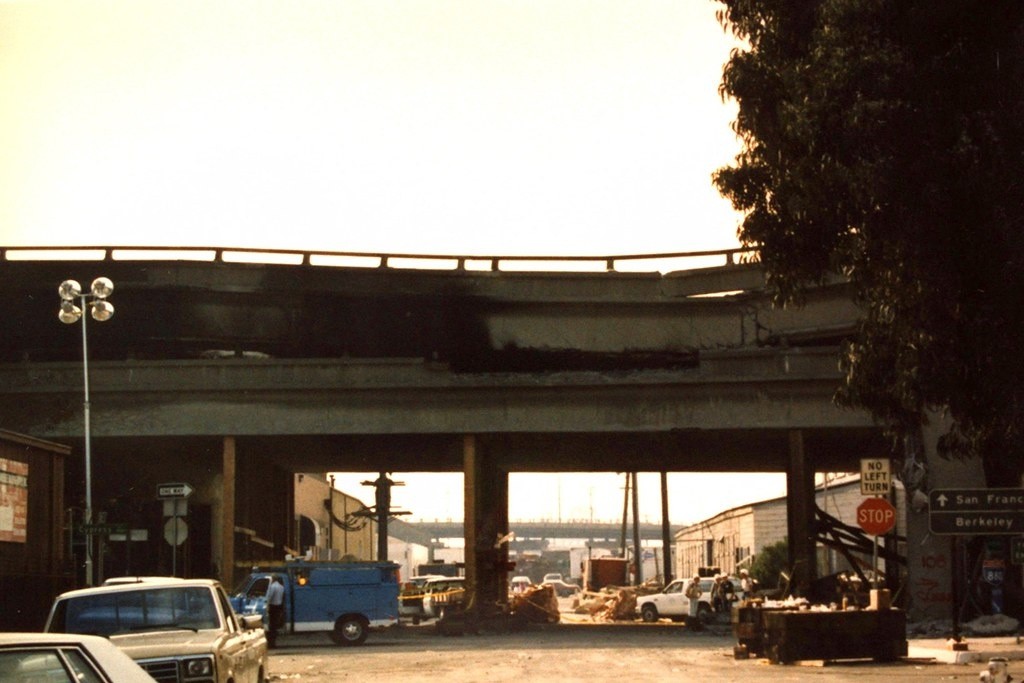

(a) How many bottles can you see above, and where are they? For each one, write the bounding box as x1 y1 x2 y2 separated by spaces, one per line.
842 596 849 611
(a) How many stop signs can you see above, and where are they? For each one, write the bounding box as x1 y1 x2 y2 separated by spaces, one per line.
856 498 896 535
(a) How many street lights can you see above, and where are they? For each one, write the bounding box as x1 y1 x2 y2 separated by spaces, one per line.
58 276 117 591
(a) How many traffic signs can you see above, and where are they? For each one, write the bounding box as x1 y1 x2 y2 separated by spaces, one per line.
927 486 1023 537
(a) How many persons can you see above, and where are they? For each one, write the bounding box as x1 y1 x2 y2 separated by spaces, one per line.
267 575 285 648
687 577 702 617
711 573 734 612
740 569 752 600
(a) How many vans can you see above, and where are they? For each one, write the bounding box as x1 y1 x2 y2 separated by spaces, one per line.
229 557 402 647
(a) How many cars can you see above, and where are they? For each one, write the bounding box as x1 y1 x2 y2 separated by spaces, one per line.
397 574 466 625
0 630 160 683
635 575 743 625
511 572 582 598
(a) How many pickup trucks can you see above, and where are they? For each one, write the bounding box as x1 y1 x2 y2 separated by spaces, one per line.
13 576 268 683
